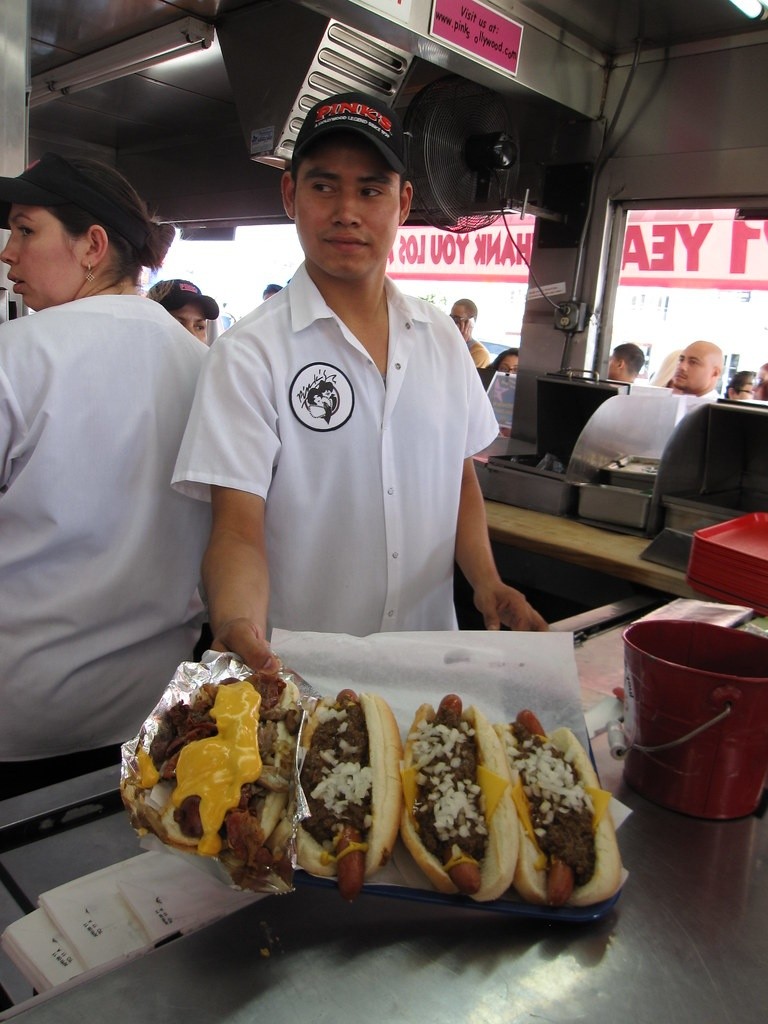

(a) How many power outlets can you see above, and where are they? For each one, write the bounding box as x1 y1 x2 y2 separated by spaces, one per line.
554 301 587 333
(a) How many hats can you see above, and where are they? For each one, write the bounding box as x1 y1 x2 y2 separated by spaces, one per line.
0 152 145 252
291 93 407 181
146 279 219 320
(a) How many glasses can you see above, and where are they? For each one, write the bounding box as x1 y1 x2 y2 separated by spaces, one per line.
499 365 518 372
449 313 467 323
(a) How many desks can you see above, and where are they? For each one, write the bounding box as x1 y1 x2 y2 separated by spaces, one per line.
478 487 745 607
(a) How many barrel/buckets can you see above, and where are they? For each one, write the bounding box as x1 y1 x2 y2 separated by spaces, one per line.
605 619 768 821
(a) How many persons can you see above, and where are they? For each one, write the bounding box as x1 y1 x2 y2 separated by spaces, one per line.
654 341 721 401
485 347 518 376
0 157 209 793
262 282 283 301
606 343 645 385
147 280 220 346
173 92 546 680
449 299 491 370
725 362 768 402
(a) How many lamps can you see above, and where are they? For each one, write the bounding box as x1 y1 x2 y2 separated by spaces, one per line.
28 15 215 108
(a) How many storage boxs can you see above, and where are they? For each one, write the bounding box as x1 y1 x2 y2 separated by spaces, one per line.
481 371 661 529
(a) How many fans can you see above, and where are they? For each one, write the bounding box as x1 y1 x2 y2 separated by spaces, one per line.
403 74 520 233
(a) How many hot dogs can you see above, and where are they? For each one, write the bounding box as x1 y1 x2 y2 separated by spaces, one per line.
164 669 622 908
